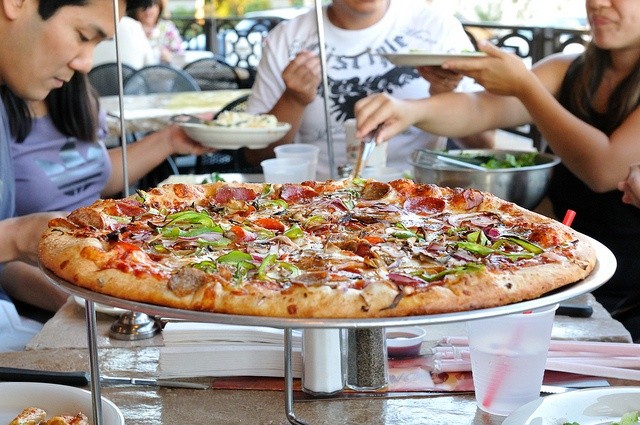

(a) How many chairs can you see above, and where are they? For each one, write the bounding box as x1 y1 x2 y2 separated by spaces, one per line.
119 62 202 172
182 57 241 92
85 60 139 98
135 149 180 196
197 93 268 177
232 63 258 88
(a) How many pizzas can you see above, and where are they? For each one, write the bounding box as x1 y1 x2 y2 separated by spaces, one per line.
35 175 598 321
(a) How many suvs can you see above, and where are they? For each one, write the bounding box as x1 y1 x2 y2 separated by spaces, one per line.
224 8 313 67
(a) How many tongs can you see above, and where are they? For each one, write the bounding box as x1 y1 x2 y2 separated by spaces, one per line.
354 125 381 180
412 151 486 170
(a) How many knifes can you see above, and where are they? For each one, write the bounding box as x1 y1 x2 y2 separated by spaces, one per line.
0 366 209 390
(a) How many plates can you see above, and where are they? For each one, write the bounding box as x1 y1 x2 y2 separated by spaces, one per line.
380 53 514 67
501 387 640 424
0 380 126 425
73 295 131 316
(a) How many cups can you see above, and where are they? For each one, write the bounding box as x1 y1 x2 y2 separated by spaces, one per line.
273 144 320 180
260 158 310 184
343 119 388 182
468 303 560 416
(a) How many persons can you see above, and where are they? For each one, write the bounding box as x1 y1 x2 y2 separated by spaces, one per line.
92 17 160 71
353 1 640 340
249 1 495 182
1 1 125 352
0 69 217 313
619 164 640 208
127 0 184 66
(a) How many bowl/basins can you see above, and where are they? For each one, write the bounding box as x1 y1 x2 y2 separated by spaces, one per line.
178 122 292 150
408 148 563 211
387 327 427 357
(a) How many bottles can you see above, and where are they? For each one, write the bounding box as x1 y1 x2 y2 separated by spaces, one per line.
300 329 344 397
347 326 388 391
